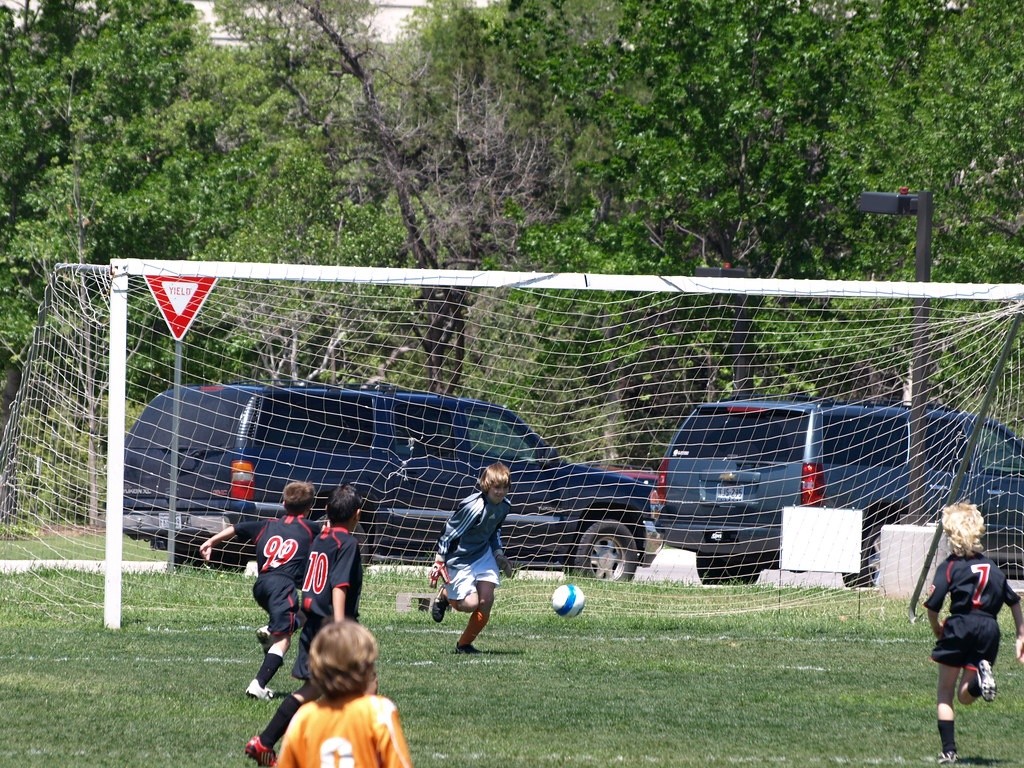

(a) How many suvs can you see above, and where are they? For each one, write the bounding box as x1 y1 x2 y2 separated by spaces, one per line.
648 394 1024 586
122 382 665 585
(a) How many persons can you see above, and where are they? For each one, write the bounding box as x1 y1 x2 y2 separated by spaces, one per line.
429 464 516 653
922 502 1024 765
201 484 415 768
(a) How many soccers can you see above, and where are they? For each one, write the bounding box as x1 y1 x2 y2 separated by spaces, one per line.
549 584 586 618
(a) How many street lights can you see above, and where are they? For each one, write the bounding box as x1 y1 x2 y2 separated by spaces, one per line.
856 187 935 525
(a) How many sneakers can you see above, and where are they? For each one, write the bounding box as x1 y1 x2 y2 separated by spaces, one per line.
938 751 959 764
246 679 274 700
456 642 480 654
245 736 278 766
977 659 997 702
256 626 274 655
432 586 449 622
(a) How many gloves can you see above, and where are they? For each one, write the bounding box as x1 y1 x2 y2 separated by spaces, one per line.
496 555 511 577
429 562 449 589
